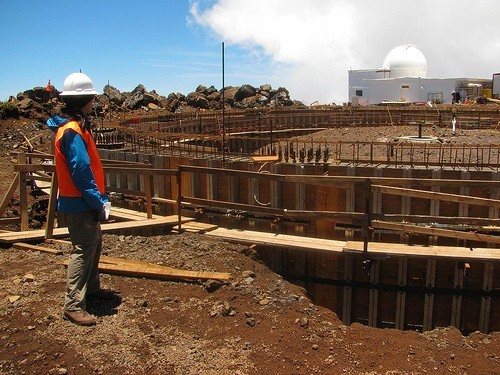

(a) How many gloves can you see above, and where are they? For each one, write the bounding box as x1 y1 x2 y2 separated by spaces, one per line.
103 202 111 221
95 205 105 221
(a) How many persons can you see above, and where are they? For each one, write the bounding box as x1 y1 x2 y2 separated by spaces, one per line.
52 73 114 327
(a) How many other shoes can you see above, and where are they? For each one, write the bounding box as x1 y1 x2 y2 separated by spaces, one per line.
62 309 96 325
88 288 115 298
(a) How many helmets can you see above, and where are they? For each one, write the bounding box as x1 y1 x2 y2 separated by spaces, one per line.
58 73 101 96
